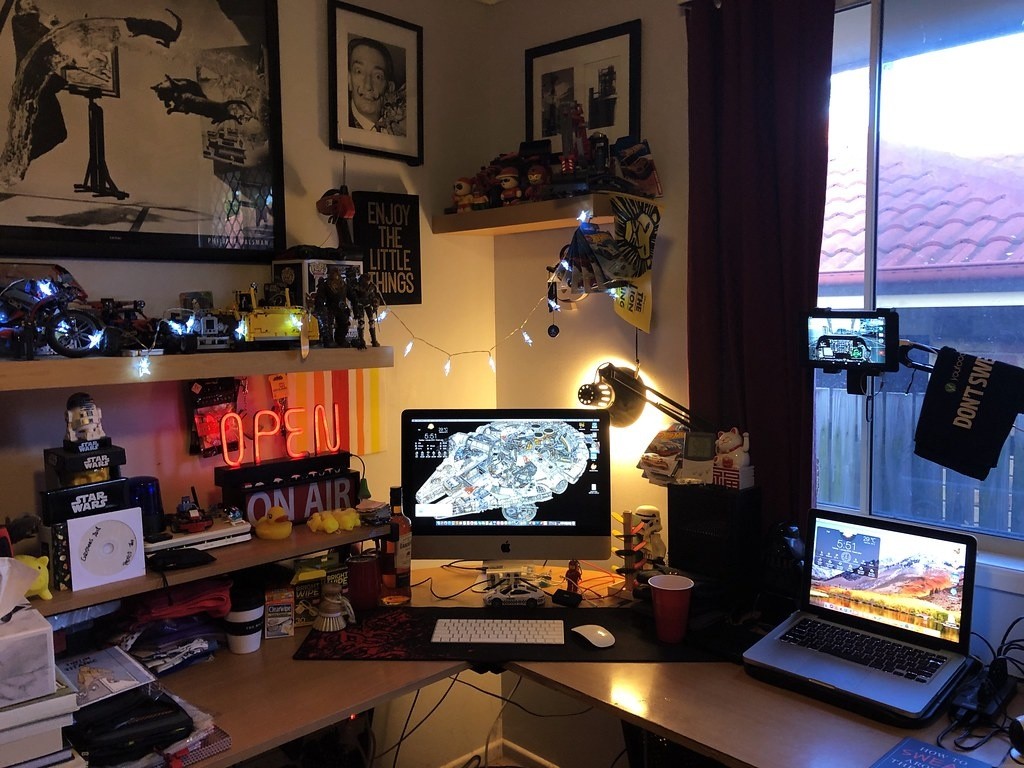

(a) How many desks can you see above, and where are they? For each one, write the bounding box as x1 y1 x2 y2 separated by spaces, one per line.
133 561 1024 768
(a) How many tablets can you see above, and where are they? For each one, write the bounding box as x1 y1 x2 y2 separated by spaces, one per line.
803 309 899 372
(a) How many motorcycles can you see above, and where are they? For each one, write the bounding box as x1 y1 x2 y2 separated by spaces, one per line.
0 265 103 359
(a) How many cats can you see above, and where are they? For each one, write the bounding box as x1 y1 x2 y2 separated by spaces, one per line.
714 426 750 468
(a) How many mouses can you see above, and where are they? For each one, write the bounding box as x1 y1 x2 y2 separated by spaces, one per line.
571 625 615 647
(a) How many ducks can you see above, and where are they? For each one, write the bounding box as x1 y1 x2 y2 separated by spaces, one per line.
255 505 292 539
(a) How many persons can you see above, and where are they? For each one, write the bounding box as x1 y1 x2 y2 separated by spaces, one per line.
348 32 396 135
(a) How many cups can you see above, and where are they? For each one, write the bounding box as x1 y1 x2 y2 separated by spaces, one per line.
648 575 694 641
223 603 264 655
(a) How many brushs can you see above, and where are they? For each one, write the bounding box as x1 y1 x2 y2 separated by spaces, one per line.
313 582 347 631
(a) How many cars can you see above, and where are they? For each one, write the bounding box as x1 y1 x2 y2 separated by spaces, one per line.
483 583 546 607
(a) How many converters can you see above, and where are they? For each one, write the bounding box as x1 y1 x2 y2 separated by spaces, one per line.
552 589 582 608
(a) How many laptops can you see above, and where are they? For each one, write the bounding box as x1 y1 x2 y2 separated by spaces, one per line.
743 508 977 719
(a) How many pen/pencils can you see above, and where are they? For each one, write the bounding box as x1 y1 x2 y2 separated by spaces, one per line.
348 545 361 560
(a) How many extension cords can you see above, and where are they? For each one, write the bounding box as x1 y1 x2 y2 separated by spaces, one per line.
950 670 990 716
978 674 1022 722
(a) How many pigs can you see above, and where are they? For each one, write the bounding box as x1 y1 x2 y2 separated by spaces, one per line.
14 556 53 602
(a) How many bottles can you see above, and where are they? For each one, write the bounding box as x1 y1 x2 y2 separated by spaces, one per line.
348 554 383 602
384 487 412 606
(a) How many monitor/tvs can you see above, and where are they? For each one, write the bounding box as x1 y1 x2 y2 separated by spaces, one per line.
400 409 612 592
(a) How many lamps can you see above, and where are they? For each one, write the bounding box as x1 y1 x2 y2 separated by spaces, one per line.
577 362 691 434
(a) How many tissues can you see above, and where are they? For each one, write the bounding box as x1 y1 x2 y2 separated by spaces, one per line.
0 556 57 709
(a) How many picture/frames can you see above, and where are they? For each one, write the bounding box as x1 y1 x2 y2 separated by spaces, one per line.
325 0 424 167
524 19 643 155
1 1 289 262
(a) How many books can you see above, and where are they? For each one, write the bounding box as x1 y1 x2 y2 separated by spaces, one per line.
149 725 232 768
0 664 89 768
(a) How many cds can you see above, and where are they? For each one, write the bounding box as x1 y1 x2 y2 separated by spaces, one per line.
79 520 137 575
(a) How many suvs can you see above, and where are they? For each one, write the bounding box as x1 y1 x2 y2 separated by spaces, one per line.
862 331 877 338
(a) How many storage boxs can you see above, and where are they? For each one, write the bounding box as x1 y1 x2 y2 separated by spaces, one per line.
0 598 57 708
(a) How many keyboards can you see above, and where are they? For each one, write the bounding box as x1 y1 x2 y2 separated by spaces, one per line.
430 619 564 644
